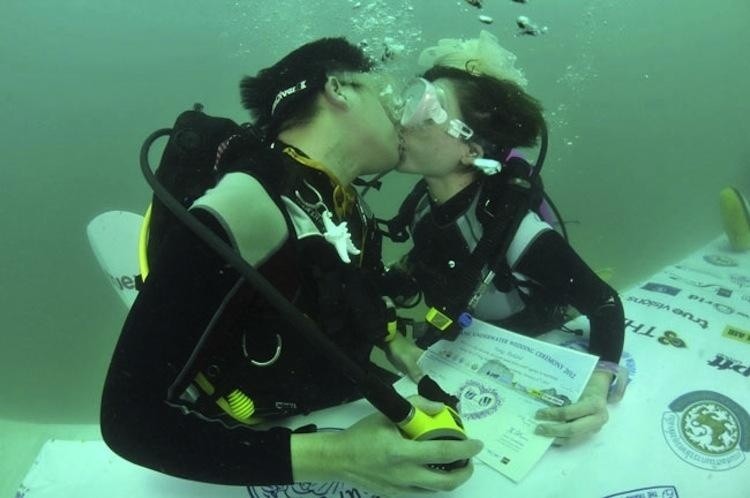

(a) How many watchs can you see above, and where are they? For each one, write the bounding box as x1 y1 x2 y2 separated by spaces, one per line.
589 359 631 405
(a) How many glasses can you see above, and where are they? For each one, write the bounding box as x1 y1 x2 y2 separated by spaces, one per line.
398 77 473 145
322 69 405 122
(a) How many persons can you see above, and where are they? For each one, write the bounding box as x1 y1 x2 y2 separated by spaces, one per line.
99 36 484 497
382 29 624 449
